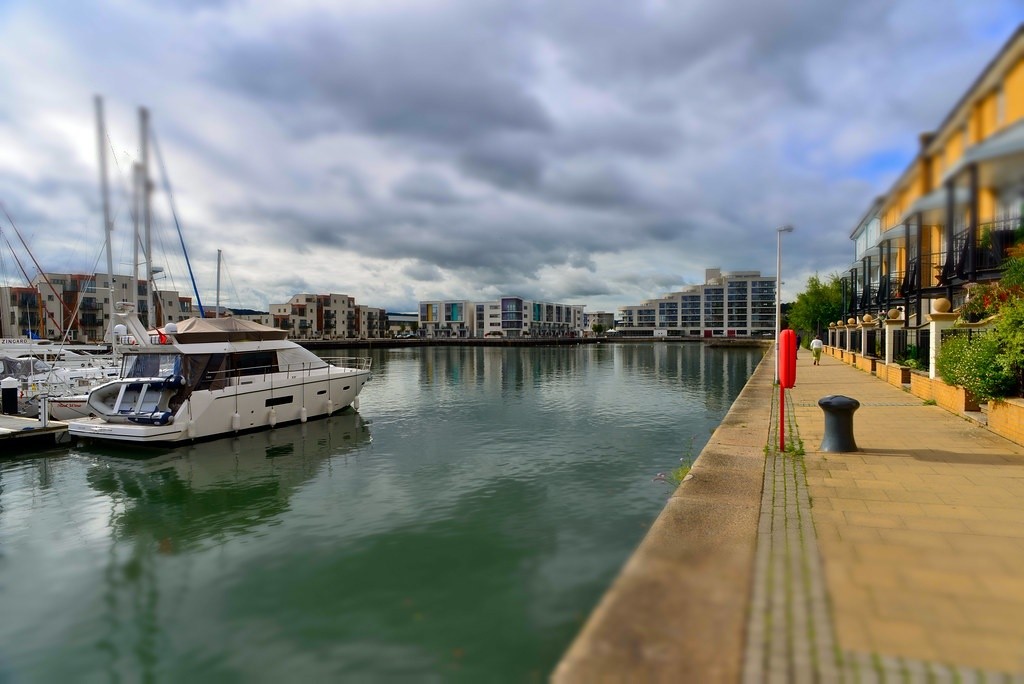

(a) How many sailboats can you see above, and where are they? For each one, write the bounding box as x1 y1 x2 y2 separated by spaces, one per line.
0 94 221 421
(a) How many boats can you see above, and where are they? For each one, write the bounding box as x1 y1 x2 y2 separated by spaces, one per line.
67 302 374 444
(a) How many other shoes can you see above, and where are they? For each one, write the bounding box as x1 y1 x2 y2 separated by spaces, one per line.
814 361 816 365
818 364 819 365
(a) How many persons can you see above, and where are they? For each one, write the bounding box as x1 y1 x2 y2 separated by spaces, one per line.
810 335 824 365
795 333 801 360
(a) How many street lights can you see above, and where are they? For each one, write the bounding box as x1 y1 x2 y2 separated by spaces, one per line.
774 225 794 384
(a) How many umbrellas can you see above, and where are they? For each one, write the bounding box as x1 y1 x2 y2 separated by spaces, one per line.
606 328 616 336
